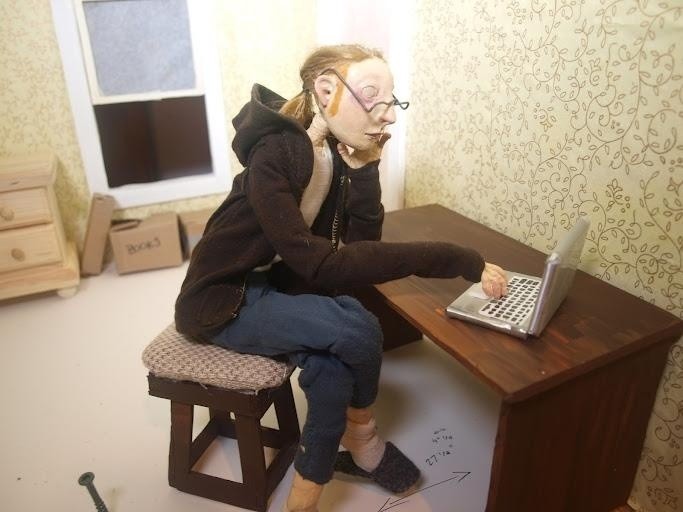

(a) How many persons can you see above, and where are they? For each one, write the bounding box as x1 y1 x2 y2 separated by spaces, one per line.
174 43 506 511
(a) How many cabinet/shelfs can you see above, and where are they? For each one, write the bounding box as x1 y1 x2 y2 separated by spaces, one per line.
0 163 80 301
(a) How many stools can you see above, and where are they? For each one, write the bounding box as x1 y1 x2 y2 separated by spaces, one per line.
142 319 302 512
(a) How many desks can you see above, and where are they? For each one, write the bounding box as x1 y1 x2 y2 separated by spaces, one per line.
333 204 683 512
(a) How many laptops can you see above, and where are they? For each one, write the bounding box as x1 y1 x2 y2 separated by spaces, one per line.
445 214 590 338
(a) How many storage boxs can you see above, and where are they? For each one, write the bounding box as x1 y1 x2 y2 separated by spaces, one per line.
81 193 217 275
